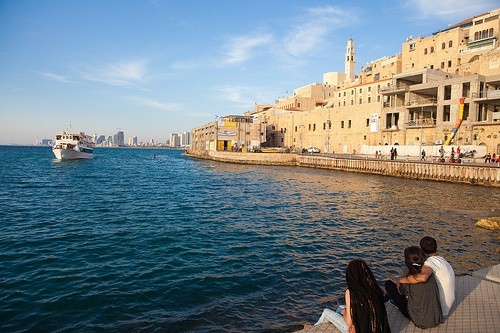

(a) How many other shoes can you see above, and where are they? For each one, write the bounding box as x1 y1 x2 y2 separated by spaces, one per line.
388 299 399 310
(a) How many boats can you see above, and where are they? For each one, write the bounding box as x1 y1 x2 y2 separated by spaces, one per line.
50 120 97 161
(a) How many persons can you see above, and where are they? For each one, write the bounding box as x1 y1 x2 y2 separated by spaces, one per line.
384 246 442 328
390 147 398 160
304 259 389 333
420 149 426 162
482 152 500 164
397 237 457 316
451 147 455 159
439 146 445 158
456 146 461 159
374 150 382 160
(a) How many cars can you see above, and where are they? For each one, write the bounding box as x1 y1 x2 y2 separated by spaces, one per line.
307 146 320 153
